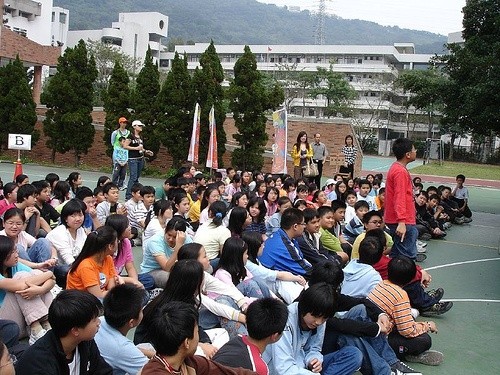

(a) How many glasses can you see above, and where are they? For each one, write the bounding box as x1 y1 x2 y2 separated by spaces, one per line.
0 354 17 368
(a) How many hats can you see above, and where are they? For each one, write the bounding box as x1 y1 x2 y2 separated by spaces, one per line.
325 179 338 186
119 117 127 123
132 120 145 127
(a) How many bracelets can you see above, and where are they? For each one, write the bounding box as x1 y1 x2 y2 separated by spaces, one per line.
52 257 59 263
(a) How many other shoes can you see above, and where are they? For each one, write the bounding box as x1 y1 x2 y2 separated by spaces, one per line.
405 349 444 365
416 245 426 252
118 186 124 190
416 239 427 247
50 283 63 300
29 328 52 346
391 369 423 375
415 254 426 262
393 361 415 373
461 216 472 222
420 233 431 240
454 217 463 224
123 184 127 189
437 231 447 237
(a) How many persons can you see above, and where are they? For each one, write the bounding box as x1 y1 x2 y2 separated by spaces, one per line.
0 165 473 375
271 134 276 163
111 117 132 191
291 131 313 180
310 133 327 190
339 135 358 179
123 120 154 202
383 137 420 264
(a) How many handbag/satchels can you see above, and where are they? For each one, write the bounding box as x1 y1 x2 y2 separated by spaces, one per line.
302 157 319 177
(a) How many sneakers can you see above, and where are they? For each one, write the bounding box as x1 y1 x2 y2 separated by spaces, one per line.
420 301 453 316
427 288 444 301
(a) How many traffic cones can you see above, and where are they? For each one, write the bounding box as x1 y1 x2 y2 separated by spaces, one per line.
13 159 23 183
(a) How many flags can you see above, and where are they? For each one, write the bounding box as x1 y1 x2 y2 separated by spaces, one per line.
206 106 219 169
272 108 285 174
187 104 201 164
